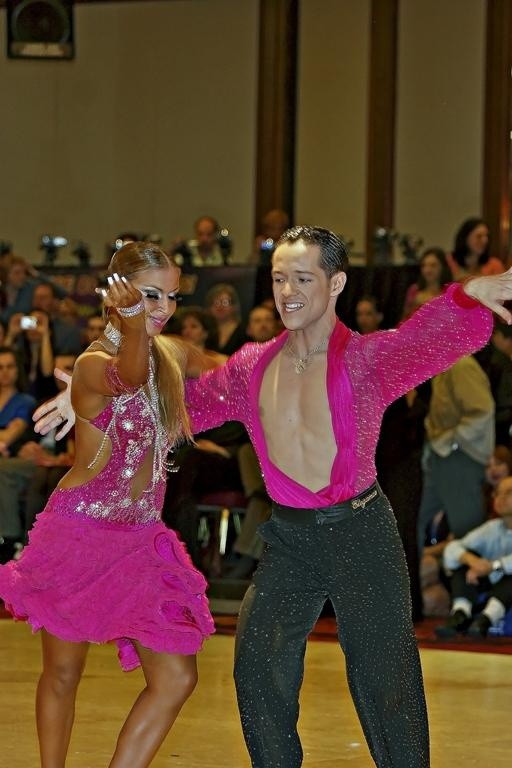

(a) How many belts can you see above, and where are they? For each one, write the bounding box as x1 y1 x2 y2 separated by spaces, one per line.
271 488 381 527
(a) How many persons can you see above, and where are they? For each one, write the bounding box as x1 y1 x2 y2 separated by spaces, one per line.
1 242 231 768
1 209 512 642
32 223 512 767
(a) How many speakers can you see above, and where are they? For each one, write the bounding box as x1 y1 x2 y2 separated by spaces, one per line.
7 2 74 61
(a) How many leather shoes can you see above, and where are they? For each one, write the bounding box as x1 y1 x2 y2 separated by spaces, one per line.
436 611 492 638
